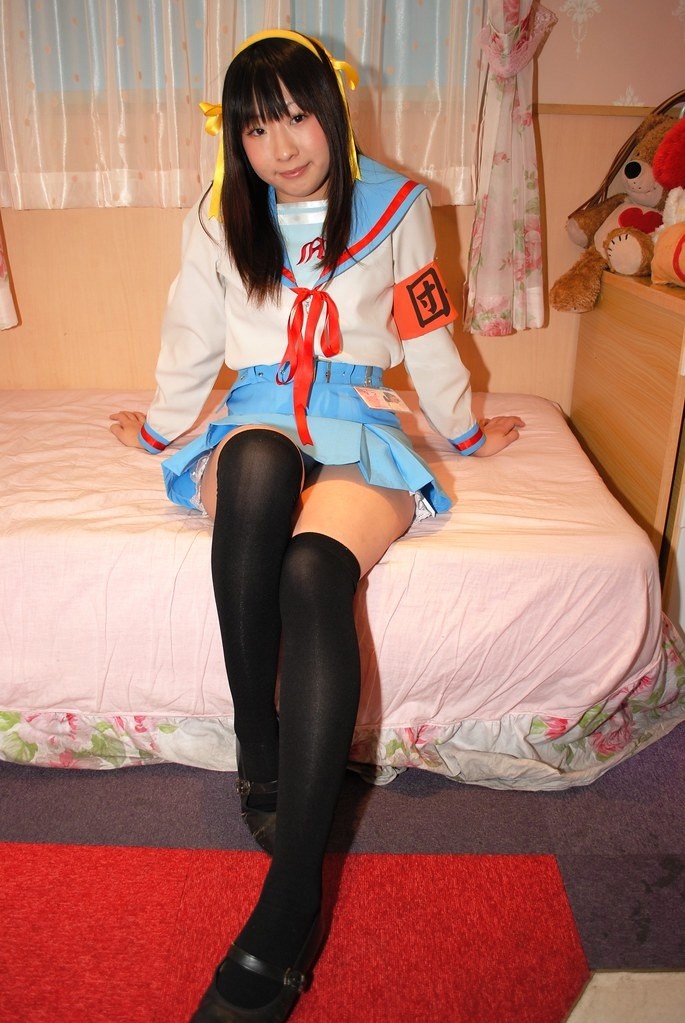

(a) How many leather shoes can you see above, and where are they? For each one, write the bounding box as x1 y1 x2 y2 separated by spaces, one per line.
189 902 324 1023
235 715 278 856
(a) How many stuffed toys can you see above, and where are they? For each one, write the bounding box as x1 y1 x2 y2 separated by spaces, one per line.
550 106 685 314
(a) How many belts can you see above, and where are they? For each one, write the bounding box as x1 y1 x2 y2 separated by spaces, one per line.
214 361 383 414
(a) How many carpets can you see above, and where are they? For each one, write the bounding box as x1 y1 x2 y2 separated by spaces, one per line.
0 842 591 1023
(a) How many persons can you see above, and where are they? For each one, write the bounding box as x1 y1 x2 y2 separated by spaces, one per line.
108 26 526 1023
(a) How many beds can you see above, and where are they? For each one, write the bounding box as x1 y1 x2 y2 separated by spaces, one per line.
0 268 685 791
